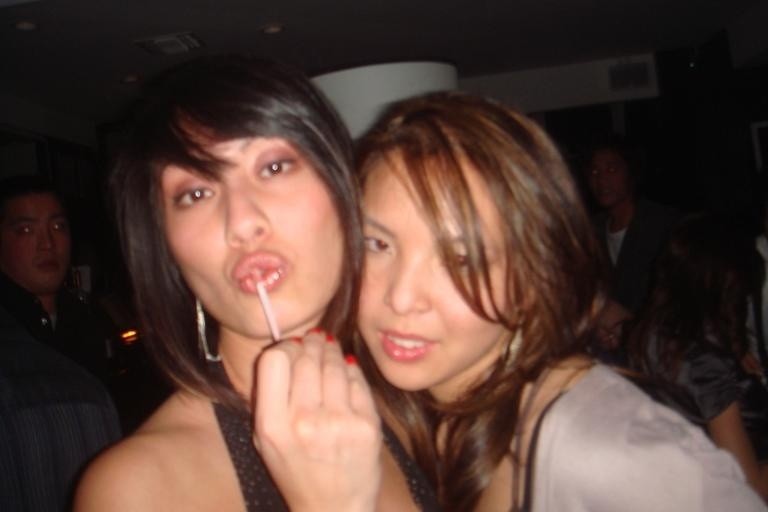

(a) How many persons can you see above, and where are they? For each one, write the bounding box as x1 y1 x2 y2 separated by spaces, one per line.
1 44 767 512
